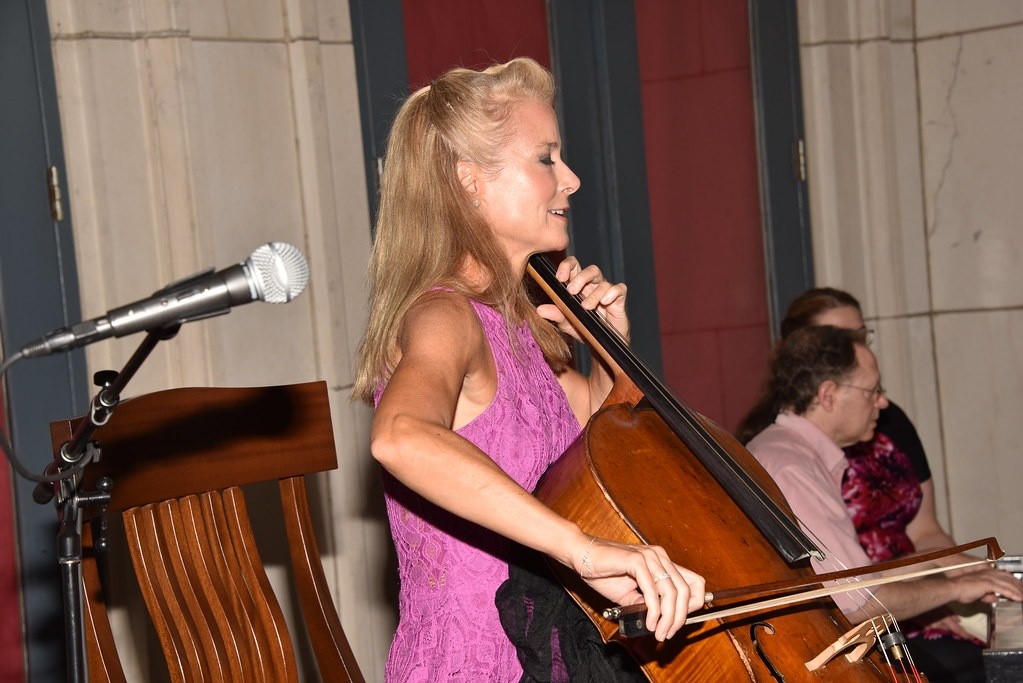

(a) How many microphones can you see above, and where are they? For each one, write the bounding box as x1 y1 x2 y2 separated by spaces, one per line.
22 243 310 358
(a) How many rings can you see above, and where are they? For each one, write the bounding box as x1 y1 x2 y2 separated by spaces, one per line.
654 575 672 584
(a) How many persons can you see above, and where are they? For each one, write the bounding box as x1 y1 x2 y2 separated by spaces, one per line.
735 286 1023 683
348 55 705 683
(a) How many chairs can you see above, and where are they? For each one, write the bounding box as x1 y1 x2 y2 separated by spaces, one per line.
48 380 365 683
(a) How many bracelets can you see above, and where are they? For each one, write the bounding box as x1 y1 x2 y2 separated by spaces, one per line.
580 536 597 581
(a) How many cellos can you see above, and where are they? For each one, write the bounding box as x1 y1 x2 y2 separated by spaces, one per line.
520 251 936 683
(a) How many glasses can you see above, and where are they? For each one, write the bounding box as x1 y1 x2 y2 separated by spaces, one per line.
833 381 888 403
855 325 876 346
